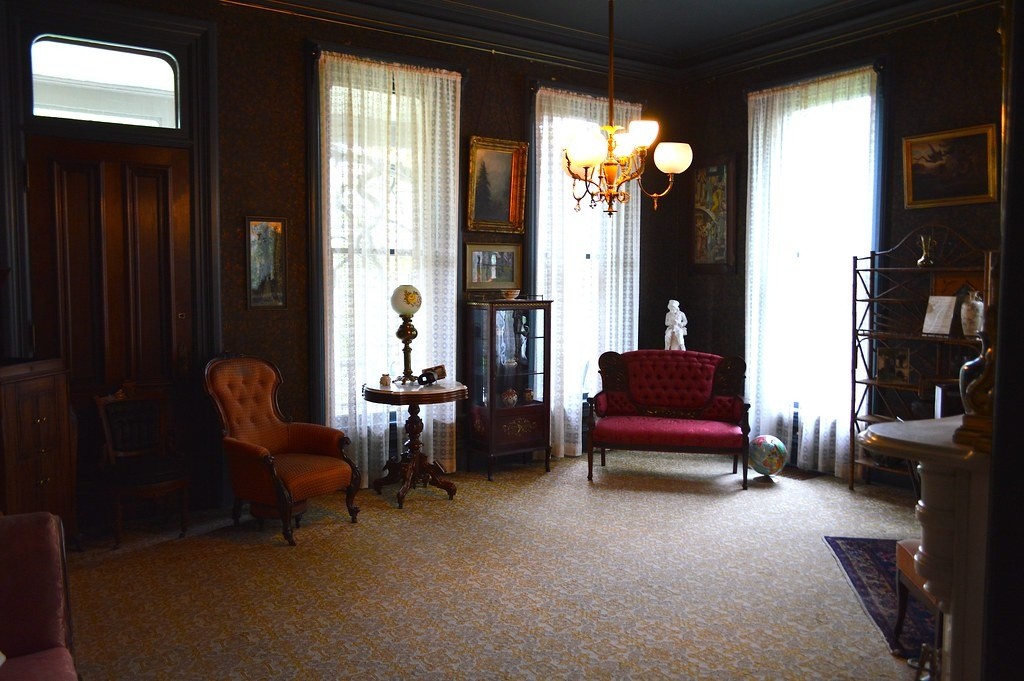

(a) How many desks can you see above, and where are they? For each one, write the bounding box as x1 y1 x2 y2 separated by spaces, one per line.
363 380 468 510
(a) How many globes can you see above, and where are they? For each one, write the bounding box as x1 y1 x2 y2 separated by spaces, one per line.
748 435 789 484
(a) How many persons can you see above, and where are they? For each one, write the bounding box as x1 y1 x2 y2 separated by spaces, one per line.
665 300 688 351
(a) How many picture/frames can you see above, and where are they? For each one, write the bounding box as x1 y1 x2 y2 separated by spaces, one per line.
876 346 909 385
466 242 524 292
902 123 997 211
468 136 529 235
245 215 289 313
690 151 738 276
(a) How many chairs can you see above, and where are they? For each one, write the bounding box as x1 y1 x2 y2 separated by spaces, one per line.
203 354 362 545
90 380 189 549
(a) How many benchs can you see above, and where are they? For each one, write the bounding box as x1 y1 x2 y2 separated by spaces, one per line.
0 509 85 681
583 349 750 491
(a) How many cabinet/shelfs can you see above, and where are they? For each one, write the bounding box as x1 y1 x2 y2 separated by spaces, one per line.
462 294 554 482
849 223 1001 492
0 358 83 553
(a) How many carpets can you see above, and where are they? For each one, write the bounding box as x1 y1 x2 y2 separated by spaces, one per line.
823 535 941 659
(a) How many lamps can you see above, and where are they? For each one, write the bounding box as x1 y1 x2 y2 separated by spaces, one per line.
392 285 422 386
552 0 693 219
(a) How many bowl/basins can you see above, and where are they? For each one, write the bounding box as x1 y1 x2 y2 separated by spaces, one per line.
501 290 520 299
